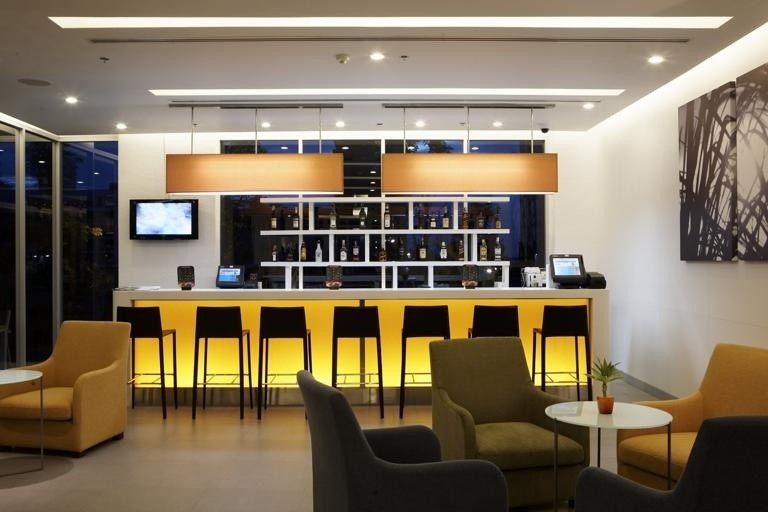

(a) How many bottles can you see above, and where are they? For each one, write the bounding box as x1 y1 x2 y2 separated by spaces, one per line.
270 204 504 262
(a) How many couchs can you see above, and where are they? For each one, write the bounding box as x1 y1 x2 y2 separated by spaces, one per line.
0 319 133 459
617 342 768 490
426 335 589 512
295 368 511 510
573 413 768 512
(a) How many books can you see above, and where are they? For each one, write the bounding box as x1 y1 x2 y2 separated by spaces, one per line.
114 285 160 291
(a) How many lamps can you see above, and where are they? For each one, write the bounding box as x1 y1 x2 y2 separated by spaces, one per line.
380 102 559 196
164 104 347 196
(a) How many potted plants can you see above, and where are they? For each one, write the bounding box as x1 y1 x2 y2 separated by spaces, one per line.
586 351 627 415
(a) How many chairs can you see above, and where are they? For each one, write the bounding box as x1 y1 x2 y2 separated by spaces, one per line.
465 304 520 339
399 305 450 418
332 305 385 419
116 305 178 420
256 305 312 420
192 305 254 420
531 304 594 400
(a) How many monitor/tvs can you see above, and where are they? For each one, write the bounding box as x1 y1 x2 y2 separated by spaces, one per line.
549 254 587 288
129 198 198 240
217 265 244 288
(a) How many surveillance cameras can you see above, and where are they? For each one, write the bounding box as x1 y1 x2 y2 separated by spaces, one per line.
540 127 549 133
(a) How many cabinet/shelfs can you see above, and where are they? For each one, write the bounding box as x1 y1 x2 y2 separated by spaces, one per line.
258 139 511 288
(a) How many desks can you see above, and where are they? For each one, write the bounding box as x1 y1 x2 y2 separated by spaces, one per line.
1 367 45 477
